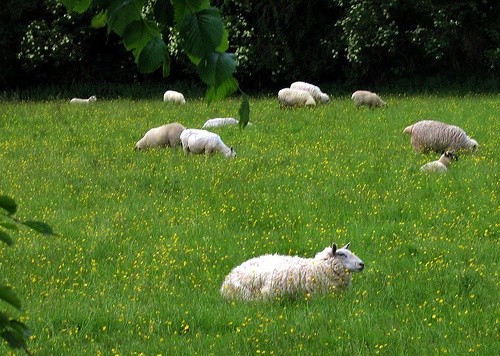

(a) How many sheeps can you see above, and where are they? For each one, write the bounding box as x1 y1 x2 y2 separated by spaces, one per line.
419 149 458 173
220 242 364 303
70 95 97 104
136 123 185 151
278 81 329 107
201 118 252 128
403 120 478 153
350 90 384 108
163 89 185 104
180 129 237 159
